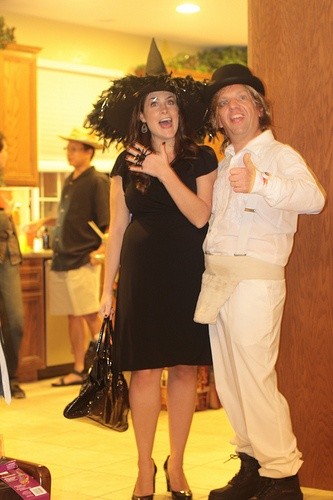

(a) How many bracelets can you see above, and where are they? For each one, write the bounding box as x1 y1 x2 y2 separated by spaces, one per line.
260 171 271 188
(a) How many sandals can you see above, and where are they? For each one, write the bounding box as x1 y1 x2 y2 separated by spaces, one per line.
51 369 87 386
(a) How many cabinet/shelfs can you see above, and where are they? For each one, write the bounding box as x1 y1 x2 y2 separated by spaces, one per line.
16 257 46 382
0 40 40 187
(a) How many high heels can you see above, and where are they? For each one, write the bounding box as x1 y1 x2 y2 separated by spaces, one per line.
132 458 157 500
163 455 192 500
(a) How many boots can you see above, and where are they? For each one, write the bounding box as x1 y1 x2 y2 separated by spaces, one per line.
252 474 303 500
208 452 265 500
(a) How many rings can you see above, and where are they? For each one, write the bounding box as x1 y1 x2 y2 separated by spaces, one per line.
132 147 154 167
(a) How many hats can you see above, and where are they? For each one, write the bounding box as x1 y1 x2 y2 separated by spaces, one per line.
58 128 104 149
207 64 265 105
84 38 217 164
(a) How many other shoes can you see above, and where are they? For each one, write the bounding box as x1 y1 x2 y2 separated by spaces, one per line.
11 384 25 398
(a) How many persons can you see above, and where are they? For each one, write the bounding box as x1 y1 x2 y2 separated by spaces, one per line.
83 38 219 500
0 133 27 399
193 63 327 500
49 126 111 388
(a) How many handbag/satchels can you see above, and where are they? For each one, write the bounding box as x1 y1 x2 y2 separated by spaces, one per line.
63 315 129 433
